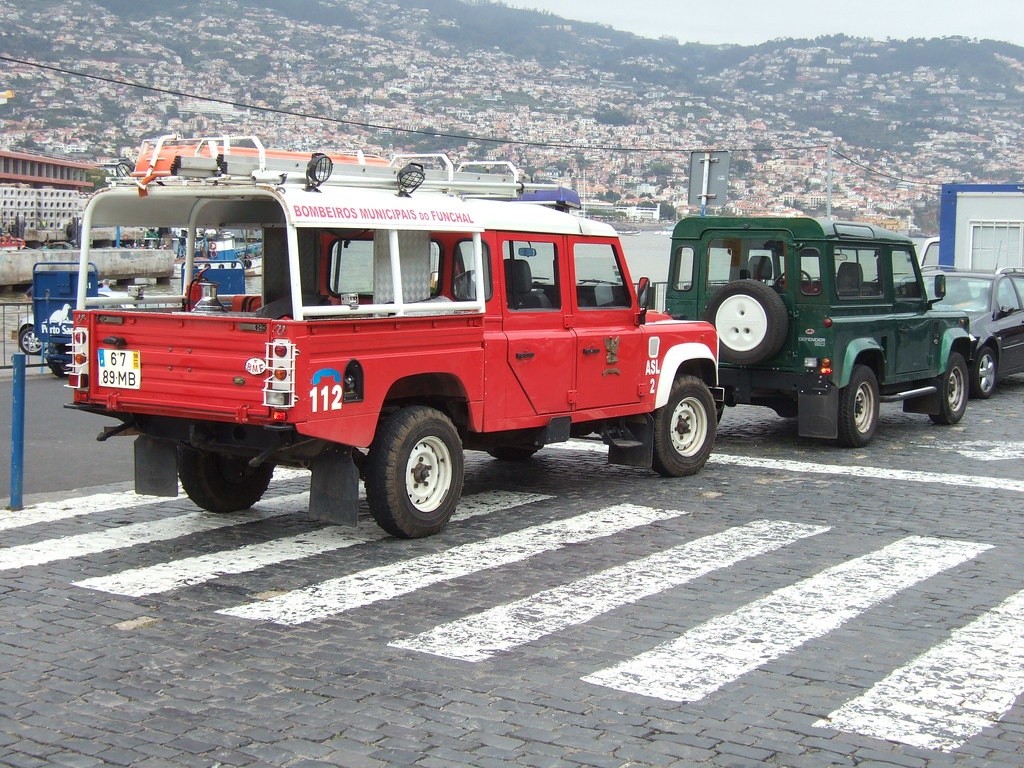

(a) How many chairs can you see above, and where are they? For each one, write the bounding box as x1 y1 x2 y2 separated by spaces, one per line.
748 256 772 286
836 262 876 296
503 258 553 309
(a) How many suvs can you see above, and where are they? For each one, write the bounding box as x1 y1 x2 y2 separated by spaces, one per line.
659 211 973 447
895 265 1024 399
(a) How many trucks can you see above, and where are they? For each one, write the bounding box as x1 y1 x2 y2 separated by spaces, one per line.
63 130 727 542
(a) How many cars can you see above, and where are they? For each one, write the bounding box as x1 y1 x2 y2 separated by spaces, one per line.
17 291 177 355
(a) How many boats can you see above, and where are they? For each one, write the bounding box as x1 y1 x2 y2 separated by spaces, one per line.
192 240 262 277
